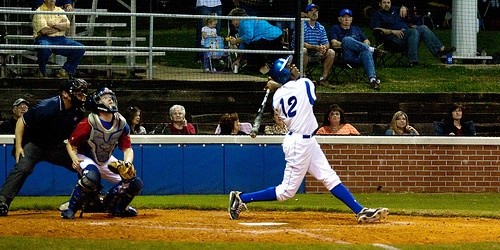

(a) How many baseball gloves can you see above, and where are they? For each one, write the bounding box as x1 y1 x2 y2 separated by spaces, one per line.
107 160 136 183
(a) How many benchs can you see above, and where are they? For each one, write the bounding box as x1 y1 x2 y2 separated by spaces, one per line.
0 7 165 80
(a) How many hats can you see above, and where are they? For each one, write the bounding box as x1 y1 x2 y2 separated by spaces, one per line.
339 9 353 16
13 98 29 106
306 3 319 11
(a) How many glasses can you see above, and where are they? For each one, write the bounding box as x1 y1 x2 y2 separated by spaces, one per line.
19 106 28 109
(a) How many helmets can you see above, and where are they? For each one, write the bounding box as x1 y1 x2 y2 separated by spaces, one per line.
89 87 118 114
269 55 293 84
61 78 89 108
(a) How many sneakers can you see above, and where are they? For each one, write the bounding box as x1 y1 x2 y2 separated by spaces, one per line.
357 207 389 224
228 191 247 220
112 205 137 216
61 200 79 219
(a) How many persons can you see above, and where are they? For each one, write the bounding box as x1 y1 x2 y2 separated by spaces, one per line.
224 8 289 76
384 111 420 135
163 105 195 134
316 104 360 135
195 0 222 74
62 88 143 220
290 4 337 88
365 1 457 66
31 0 86 79
0 78 103 217
434 102 475 136
120 107 147 134
330 9 387 90
215 114 248 135
264 108 274 139
0 98 30 135
200 16 225 73
229 55 389 224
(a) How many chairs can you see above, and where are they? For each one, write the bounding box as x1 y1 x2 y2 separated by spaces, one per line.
307 52 340 85
204 35 233 73
332 46 378 85
373 29 408 68
435 120 476 137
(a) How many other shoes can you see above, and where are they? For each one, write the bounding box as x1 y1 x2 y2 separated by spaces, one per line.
0 202 8 216
439 46 456 57
409 61 425 68
320 79 333 88
369 80 380 90
372 47 388 58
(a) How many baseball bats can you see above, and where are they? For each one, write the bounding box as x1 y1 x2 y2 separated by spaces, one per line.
250 89 270 138
